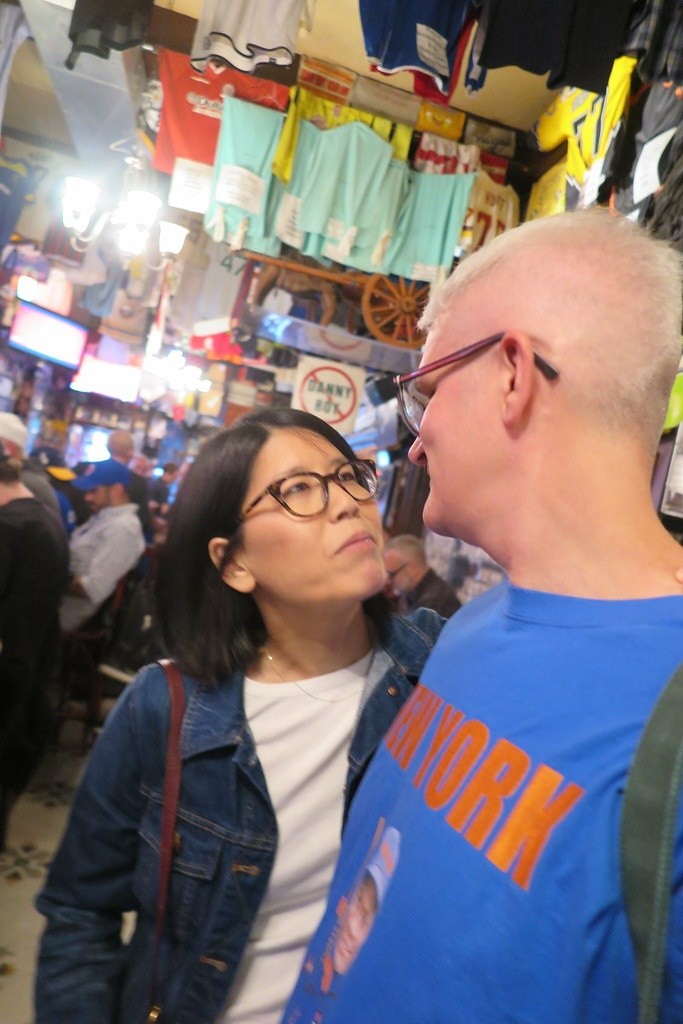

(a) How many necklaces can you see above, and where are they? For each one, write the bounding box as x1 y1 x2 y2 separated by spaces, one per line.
265 647 365 703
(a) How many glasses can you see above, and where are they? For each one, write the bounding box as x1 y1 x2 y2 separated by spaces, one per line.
390 333 560 438
224 458 379 537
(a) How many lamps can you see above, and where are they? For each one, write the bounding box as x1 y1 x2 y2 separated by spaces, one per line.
59 135 190 272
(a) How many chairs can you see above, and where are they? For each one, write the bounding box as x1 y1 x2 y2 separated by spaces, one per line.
40 577 126 750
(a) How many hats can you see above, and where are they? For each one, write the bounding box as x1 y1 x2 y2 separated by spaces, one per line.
0 411 28 460
30 446 76 481
70 460 131 492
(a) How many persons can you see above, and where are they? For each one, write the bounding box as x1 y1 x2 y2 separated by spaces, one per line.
285 207 683 1024
34 409 448 1023
0 412 178 879
385 534 462 620
332 827 401 976
